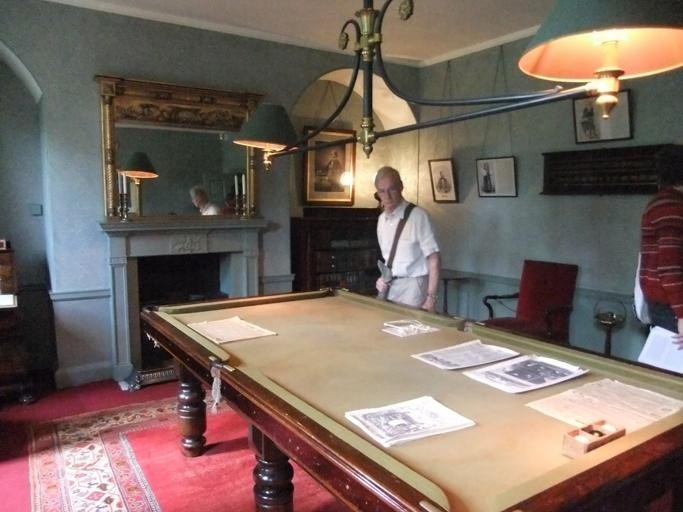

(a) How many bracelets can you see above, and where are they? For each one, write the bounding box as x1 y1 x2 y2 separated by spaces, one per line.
426 293 438 301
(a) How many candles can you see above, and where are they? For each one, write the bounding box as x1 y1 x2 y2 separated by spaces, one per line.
122 174 127 193
241 174 246 195
234 175 239 195
117 175 122 193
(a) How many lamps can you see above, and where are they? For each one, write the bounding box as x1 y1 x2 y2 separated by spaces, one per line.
232 0 683 174
114 149 160 187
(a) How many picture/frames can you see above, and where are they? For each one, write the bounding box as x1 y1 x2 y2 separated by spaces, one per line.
571 88 634 146
427 155 518 203
301 124 357 207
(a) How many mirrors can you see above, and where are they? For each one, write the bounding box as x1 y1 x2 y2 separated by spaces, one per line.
92 74 267 222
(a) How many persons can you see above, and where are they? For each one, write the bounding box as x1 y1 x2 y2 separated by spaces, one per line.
188 186 222 216
373 165 440 312
480 163 491 191
317 150 345 191
636 149 682 352
435 170 449 191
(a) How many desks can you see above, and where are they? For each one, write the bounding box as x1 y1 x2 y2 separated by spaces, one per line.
138 284 683 512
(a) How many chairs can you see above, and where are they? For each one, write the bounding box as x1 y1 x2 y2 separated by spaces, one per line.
479 257 579 346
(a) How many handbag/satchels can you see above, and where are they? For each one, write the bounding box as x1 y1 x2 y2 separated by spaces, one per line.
634 252 651 325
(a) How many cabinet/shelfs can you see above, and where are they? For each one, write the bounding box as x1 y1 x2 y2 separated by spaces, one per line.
289 215 385 295
